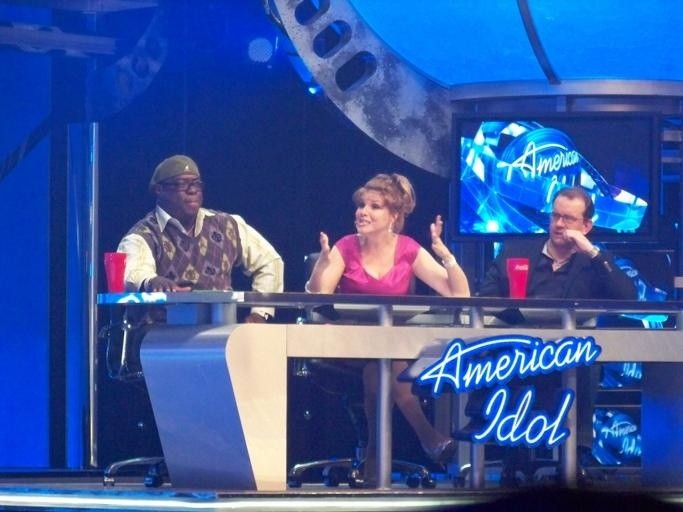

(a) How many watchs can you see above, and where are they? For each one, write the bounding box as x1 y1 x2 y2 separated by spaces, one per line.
257 310 271 320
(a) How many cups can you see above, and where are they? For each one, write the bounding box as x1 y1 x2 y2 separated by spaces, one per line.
102 251 128 293
505 256 530 301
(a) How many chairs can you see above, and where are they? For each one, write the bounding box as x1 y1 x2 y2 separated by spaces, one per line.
453 444 589 488
103 310 169 486
288 251 437 491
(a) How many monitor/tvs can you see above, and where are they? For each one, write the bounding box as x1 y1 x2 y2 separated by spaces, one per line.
450 112 661 249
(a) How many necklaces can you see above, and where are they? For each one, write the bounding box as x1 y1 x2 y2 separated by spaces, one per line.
546 239 577 272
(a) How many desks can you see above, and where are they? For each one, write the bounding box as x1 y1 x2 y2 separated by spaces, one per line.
96 288 682 491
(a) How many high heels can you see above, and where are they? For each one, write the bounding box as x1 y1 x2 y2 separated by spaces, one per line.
364 462 377 482
422 436 455 470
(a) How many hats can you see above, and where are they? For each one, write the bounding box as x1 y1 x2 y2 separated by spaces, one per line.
148 154 199 186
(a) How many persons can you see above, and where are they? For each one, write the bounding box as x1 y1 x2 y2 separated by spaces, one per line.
306 169 473 472
478 186 638 479
115 153 310 470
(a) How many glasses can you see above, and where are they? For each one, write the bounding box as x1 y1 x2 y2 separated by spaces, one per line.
550 211 583 224
162 180 205 193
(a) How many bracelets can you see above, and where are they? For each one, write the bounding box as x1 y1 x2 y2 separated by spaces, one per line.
441 254 457 270
585 247 599 257
